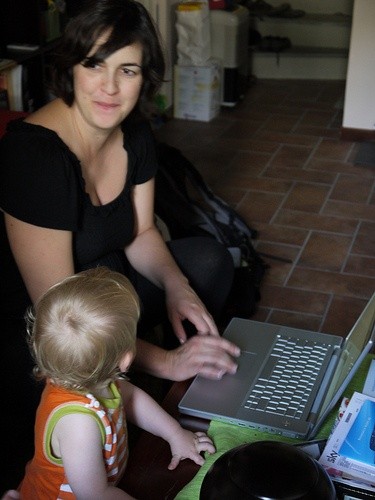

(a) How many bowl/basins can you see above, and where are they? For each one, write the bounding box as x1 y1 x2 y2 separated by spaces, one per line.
198 439 336 500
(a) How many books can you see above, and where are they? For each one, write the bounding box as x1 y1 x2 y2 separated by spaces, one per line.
316 392 375 500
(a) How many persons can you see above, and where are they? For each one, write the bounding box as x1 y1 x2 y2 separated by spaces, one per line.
0 0 242 383
0 266 216 500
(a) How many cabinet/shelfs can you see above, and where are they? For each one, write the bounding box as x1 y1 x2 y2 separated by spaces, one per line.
0 33 63 113
247 0 353 58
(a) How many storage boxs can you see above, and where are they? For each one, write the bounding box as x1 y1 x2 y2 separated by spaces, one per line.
173 62 222 121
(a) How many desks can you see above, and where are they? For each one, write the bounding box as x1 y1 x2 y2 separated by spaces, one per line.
116 380 206 500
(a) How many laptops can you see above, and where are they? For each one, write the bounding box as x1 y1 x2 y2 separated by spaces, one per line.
177 288 375 441
